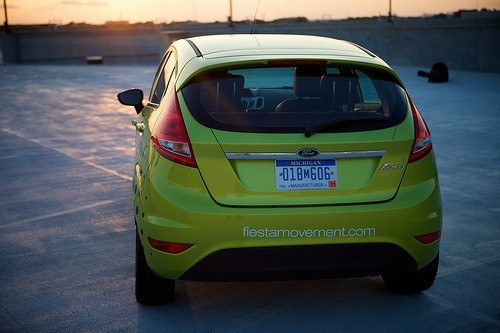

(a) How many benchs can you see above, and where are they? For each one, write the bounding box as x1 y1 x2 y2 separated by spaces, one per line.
205 73 362 120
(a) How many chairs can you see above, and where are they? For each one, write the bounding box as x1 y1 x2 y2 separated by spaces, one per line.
275 66 329 113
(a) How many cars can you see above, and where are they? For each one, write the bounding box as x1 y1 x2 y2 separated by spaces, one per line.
116 34 443 306
